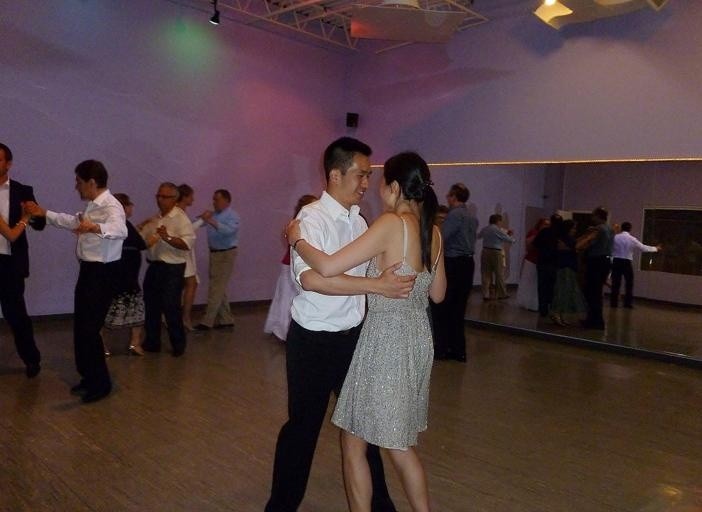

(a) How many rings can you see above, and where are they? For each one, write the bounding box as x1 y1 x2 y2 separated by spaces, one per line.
284 234 287 238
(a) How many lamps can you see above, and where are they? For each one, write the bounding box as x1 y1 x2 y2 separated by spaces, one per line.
204 0 224 25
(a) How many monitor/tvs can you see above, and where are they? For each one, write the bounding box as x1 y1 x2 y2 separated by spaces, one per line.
638 206 699 276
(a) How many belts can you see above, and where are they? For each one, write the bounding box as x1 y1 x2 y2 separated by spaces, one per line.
210 246 237 253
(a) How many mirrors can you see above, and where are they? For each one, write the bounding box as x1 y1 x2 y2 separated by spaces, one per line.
524 155 701 366
360 160 525 339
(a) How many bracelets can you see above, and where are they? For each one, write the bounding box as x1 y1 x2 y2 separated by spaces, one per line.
293 238 306 250
17 220 28 227
94 223 100 234
151 233 160 240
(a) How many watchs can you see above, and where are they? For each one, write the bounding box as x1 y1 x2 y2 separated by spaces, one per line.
166 236 173 242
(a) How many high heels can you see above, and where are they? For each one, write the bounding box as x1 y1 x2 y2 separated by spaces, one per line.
126 345 145 356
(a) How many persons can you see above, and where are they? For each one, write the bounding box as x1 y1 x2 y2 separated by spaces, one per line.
431 201 451 224
192 190 241 332
139 182 195 359
263 136 419 512
174 183 211 333
0 207 40 244
99 190 168 358
476 205 662 334
23 159 129 404
1 144 44 379
434 181 479 361
261 193 318 346
282 149 448 512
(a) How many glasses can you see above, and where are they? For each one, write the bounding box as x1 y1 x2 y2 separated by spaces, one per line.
126 202 133 207
155 193 173 199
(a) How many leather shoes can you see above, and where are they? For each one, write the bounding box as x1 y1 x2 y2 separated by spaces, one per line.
213 324 234 328
193 324 210 330
26 364 40 378
172 344 186 355
71 385 82 395
81 392 98 402
